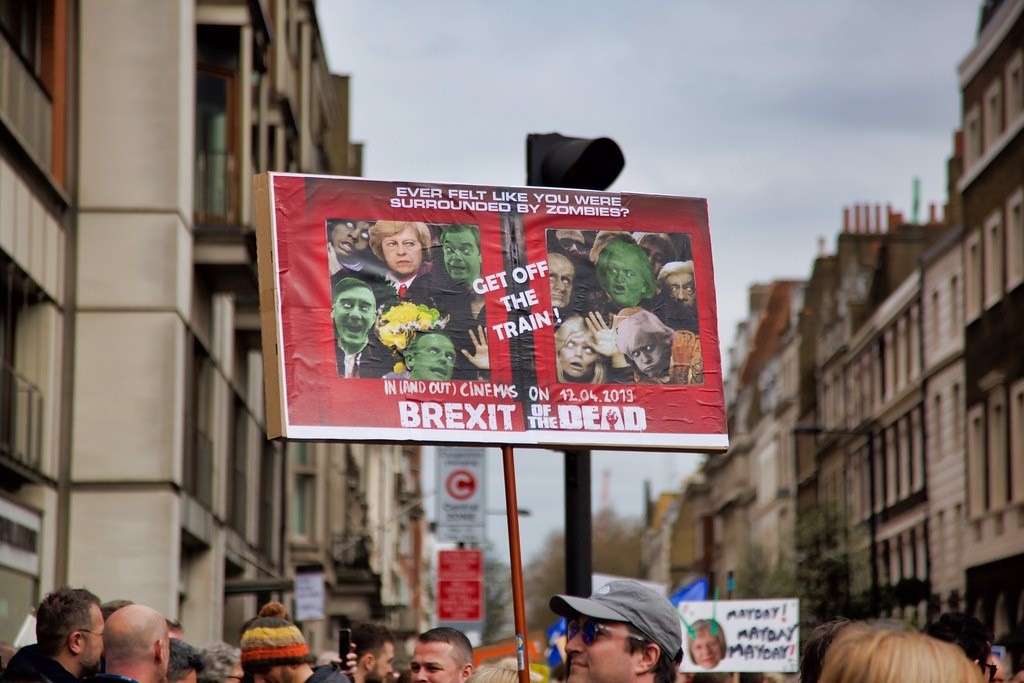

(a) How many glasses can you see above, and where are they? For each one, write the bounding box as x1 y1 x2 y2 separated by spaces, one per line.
985 663 997 680
567 618 645 646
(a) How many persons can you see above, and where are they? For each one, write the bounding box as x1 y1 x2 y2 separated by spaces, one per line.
327 219 704 389
0 578 1024 683
687 618 729 669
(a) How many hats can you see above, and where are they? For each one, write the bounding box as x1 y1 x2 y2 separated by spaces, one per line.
240 602 316 671
550 579 682 660
334 277 371 294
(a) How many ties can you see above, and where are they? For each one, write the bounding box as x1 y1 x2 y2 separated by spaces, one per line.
398 284 407 300
